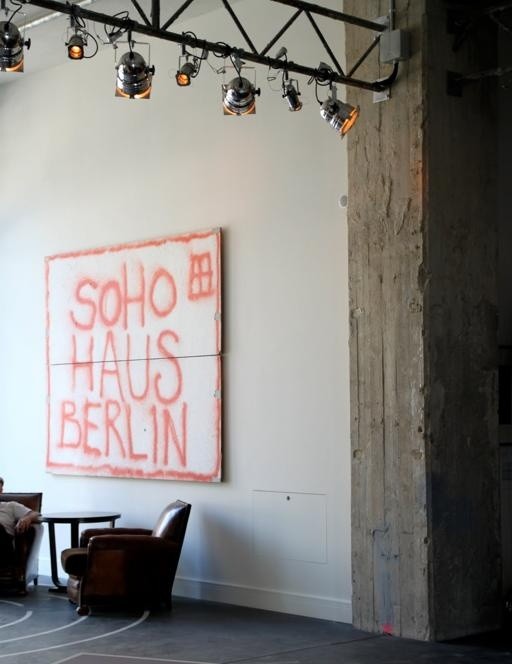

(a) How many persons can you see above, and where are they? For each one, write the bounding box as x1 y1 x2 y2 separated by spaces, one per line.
1 477 42 568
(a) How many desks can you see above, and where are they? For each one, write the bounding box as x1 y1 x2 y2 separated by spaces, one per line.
37 511 121 595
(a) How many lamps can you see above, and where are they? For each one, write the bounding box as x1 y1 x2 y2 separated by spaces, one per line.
0 0 361 137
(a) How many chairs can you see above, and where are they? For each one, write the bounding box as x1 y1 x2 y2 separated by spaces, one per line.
60 498 191 617
0 490 42 602
